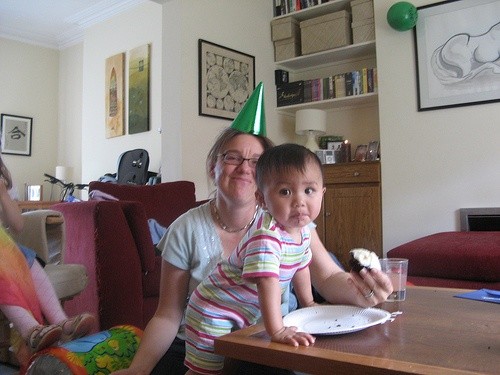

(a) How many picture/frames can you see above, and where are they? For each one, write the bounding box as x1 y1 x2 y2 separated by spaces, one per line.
414 0 500 113
0 113 33 157
198 38 256 121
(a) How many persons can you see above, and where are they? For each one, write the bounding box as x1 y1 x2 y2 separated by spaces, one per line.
184 143 326 375
107 81 394 375
0 228 95 351
0 155 25 231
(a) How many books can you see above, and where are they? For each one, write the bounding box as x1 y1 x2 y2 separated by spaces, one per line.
303 68 377 103
273 0 330 17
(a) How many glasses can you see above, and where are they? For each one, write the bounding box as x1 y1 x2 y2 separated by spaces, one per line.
217 154 258 167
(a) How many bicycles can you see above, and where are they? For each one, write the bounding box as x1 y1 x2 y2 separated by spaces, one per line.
43 173 90 203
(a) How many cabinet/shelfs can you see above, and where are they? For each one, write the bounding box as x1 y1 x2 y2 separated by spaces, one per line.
314 159 383 272
270 0 379 118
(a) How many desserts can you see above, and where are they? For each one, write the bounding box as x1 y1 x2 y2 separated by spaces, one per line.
348 248 381 277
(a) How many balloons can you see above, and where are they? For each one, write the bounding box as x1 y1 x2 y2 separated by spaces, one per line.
387 1 419 33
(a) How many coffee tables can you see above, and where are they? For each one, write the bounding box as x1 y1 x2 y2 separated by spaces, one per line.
215 285 500 375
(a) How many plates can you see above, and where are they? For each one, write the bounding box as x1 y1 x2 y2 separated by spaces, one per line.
282 305 393 333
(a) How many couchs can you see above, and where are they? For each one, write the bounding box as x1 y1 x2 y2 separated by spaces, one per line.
46 178 212 335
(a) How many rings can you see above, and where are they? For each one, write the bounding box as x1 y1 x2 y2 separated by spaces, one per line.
364 292 374 300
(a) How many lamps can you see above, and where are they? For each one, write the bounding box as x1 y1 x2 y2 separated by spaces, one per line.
294 108 328 153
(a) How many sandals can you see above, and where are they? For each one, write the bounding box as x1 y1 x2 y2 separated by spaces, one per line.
25 324 62 355
57 313 95 342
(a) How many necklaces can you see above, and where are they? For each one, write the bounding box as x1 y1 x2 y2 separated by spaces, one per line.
213 202 258 232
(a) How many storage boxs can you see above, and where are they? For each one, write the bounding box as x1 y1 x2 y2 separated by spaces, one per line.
271 0 376 62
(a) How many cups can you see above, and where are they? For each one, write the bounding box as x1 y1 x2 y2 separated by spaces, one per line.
379 258 409 303
27 185 43 201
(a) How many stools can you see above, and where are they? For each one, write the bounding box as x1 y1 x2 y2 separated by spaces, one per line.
387 231 500 290
